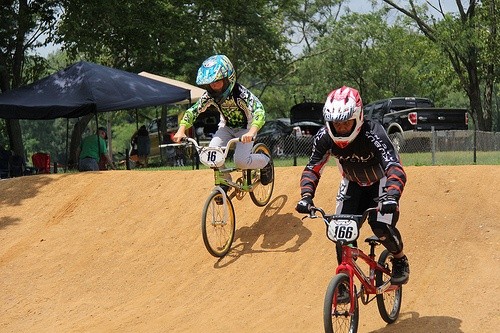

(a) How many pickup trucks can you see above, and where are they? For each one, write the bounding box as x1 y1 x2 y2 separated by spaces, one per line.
363 97 469 134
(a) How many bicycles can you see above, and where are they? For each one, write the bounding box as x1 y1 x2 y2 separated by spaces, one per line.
295 200 403 333
171 134 275 258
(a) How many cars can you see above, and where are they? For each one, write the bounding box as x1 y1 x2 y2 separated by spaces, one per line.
147 108 189 157
253 118 327 147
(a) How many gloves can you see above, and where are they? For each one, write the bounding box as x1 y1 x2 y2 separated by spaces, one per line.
296 193 315 214
378 189 401 216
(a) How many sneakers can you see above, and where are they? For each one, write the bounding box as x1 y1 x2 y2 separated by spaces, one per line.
260 153 274 185
335 285 357 302
213 183 232 202
389 253 409 284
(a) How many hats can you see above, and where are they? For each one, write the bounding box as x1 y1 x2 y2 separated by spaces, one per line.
99 127 108 140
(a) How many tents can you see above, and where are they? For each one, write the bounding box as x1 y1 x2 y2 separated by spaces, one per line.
0 62 191 170
137 71 208 106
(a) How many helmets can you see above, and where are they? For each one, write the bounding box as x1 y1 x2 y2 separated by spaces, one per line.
196 54 236 104
323 86 364 149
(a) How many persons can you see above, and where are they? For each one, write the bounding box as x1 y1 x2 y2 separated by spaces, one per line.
172 54 273 201
78 127 118 171
131 125 154 168
296 86 410 302
